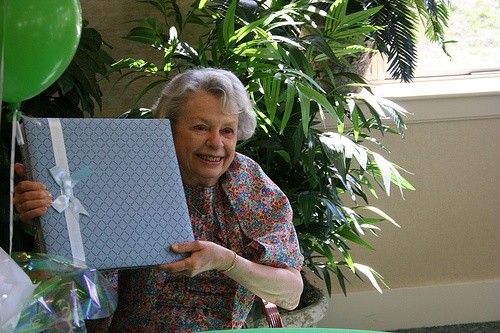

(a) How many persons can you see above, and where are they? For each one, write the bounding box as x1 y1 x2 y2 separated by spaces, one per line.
14 67 306 333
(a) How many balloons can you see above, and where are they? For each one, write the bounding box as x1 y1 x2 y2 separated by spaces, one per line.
1 1 85 122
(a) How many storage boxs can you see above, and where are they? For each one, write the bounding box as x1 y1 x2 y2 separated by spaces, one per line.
15 115 196 271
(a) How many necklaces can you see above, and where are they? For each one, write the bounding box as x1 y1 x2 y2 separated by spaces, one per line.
185 194 218 218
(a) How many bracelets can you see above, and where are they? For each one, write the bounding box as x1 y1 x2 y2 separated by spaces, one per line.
219 251 238 274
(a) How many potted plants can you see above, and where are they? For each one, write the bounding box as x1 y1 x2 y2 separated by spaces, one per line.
281 1 458 94
